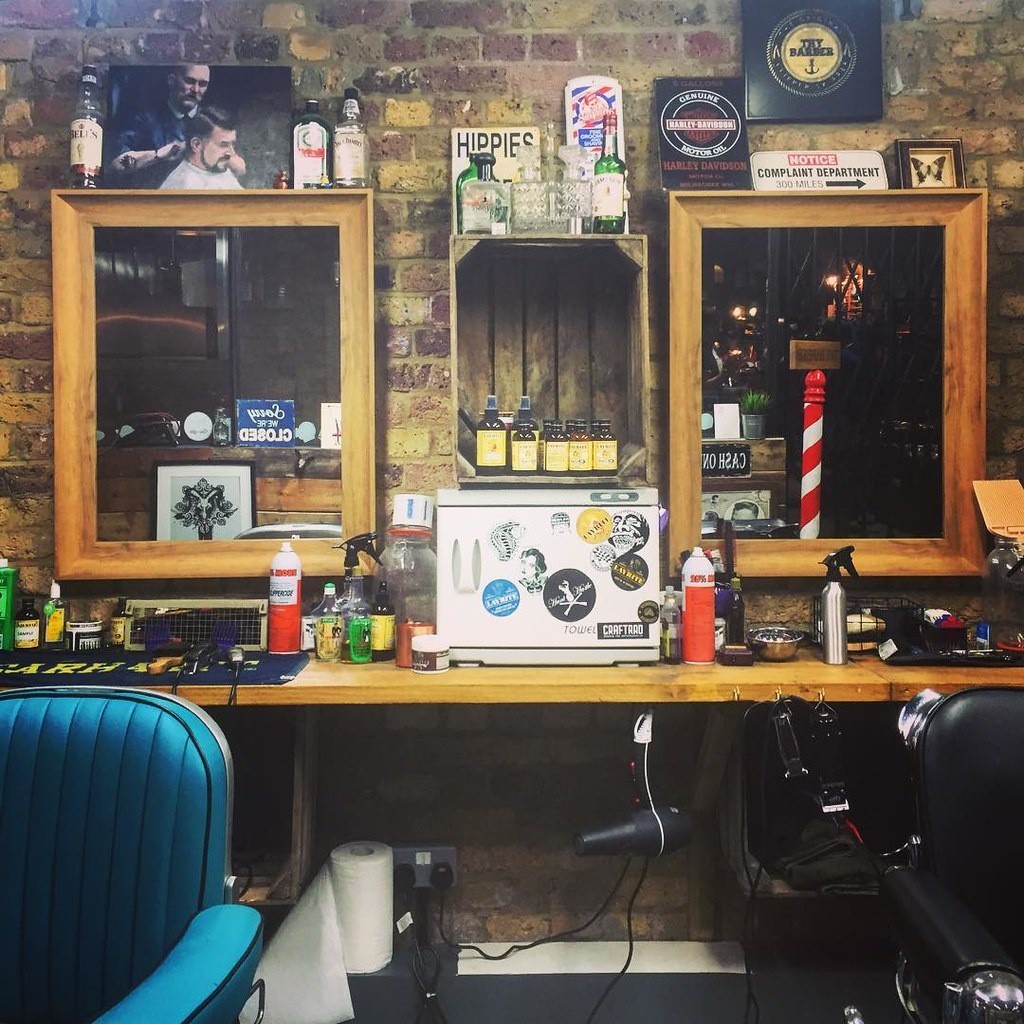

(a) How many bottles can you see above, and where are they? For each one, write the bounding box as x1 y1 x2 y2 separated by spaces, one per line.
981 537 1024 657
456 145 590 235
592 114 627 234
371 527 437 662
475 393 617 477
70 65 103 188
660 586 681 664
0 559 69 652
725 578 745 646
269 542 302 654
291 88 371 191
340 567 372 664
681 547 715 665
110 597 129 653
310 583 343 663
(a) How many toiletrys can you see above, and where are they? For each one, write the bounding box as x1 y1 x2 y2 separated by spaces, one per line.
0 556 19 652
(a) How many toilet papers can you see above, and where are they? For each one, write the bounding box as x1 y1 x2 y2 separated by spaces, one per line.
237 840 399 1024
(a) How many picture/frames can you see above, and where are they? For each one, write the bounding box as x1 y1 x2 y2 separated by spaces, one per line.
152 460 255 540
896 138 967 189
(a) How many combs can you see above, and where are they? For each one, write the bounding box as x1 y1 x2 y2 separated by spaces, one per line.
721 519 737 584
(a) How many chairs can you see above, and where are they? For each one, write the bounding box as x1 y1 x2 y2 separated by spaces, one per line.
0 685 266 1024
845 683 1024 1024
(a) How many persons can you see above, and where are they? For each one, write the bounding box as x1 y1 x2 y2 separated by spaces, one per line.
159 104 246 189
104 64 246 190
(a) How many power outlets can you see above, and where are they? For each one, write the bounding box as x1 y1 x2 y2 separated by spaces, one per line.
393 847 458 888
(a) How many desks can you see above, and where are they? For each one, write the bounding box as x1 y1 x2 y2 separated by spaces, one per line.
2 646 1024 706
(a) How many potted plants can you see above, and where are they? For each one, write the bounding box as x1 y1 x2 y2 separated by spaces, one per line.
739 388 776 439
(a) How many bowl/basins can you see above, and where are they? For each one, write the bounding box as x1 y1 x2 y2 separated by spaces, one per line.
745 627 804 661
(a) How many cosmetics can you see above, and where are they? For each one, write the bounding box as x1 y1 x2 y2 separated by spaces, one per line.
269 543 449 673
476 396 617 476
0 558 128 653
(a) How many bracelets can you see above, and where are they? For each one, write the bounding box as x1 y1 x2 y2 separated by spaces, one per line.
155 149 161 163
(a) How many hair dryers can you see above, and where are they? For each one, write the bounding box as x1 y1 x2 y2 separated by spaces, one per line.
570 759 691 860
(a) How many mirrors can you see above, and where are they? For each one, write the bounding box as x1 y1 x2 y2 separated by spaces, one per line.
669 188 988 578
51 188 377 577
